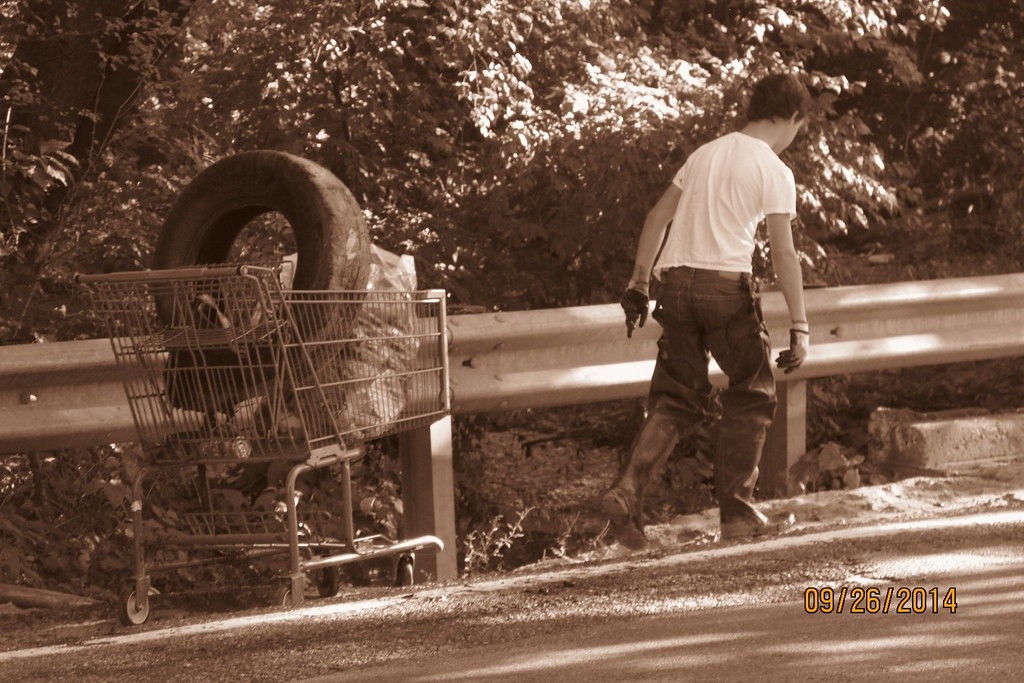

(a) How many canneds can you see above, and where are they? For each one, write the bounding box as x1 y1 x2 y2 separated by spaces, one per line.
199 438 252 458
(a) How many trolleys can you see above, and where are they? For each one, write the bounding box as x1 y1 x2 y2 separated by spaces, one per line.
72 264 453 625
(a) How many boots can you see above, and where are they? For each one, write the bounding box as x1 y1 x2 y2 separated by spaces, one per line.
711 427 795 541
601 412 679 551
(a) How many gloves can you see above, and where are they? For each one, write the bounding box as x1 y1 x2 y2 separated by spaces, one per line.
620 264 651 338
776 321 809 374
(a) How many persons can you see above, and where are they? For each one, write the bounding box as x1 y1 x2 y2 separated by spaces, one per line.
600 70 815 554
